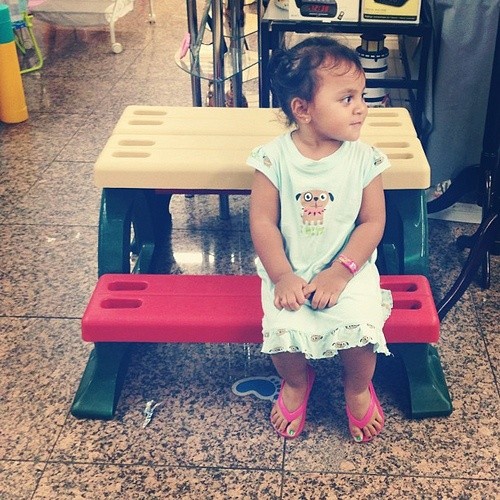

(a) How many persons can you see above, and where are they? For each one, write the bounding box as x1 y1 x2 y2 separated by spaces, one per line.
247 36 394 442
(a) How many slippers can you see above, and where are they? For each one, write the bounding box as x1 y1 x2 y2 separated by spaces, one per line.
269 365 315 438
345 379 385 443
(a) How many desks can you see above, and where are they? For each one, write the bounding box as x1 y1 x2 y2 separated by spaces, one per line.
70 105 453 420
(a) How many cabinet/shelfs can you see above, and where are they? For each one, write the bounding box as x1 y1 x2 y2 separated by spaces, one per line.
258 0 434 137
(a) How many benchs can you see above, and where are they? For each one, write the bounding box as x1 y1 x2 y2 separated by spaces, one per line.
81 274 440 344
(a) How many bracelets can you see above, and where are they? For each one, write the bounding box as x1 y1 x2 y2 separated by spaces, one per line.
336 254 358 277
